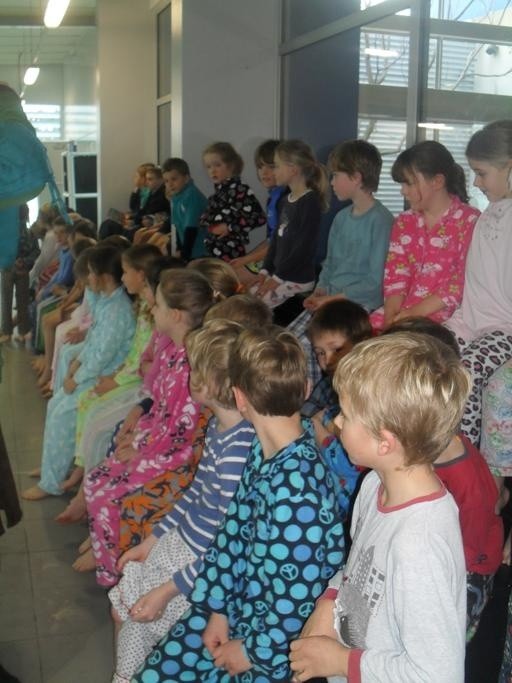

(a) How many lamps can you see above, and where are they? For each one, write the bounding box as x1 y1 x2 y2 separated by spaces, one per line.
17 0 40 98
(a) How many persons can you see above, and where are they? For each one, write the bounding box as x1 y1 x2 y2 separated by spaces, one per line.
124 120 512 496
1 76 54 272
10 194 512 683
